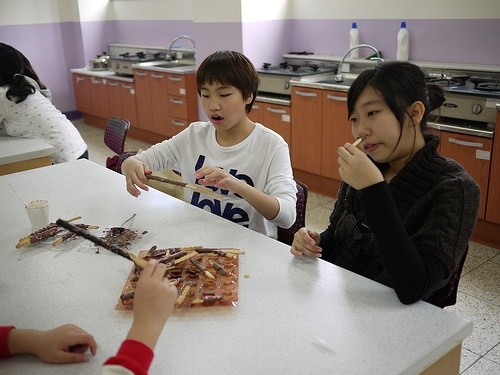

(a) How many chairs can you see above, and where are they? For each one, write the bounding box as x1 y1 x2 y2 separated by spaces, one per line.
104 117 137 174
278 180 309 246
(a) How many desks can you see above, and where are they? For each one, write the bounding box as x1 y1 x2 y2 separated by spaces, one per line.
0 158 473 375
0 129 56 177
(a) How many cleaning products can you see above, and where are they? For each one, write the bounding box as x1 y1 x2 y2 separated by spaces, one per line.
396 21 410 62
348 22 359 59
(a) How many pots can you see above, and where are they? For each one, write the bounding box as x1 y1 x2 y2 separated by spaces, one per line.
88 52 113 70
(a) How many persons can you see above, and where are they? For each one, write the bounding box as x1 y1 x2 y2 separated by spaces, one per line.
121 49 298 240
0 42 89 164
0 258 178 375
290 61 480 309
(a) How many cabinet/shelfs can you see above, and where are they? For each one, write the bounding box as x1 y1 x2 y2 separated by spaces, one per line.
72 68 199 145
430 110 500 250
247 86 363 199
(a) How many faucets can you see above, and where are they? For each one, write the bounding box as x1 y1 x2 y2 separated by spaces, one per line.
164 36 195 61
334 44 381 83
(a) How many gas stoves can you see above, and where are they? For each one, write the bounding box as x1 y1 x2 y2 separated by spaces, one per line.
253 59 338 98
109 50 171 77
420 71 500 130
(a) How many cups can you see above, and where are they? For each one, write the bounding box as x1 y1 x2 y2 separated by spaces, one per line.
24 200 49 229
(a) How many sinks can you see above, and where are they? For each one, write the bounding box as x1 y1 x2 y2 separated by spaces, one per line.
133 61 195 74
289 74 355 92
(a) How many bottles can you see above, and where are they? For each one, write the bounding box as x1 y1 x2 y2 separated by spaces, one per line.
396 21 410 62
349 22 358 59
